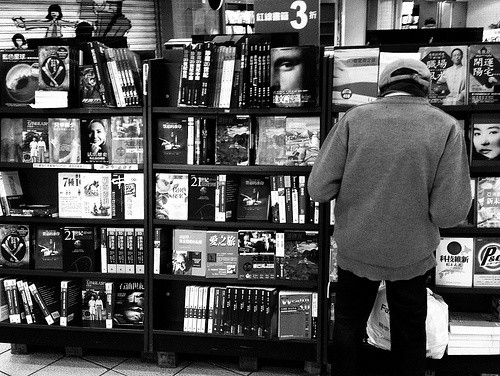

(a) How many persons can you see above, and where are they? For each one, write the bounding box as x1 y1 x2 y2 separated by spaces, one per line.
307 58 473 376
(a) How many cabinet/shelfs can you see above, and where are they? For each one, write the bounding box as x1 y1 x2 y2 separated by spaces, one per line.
147 39 328 371
327 29 500 375
1 39 149 361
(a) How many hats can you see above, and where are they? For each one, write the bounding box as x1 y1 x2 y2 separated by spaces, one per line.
380 56 431 90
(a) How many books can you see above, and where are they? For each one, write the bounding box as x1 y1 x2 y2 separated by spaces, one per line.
0 45 499 356
